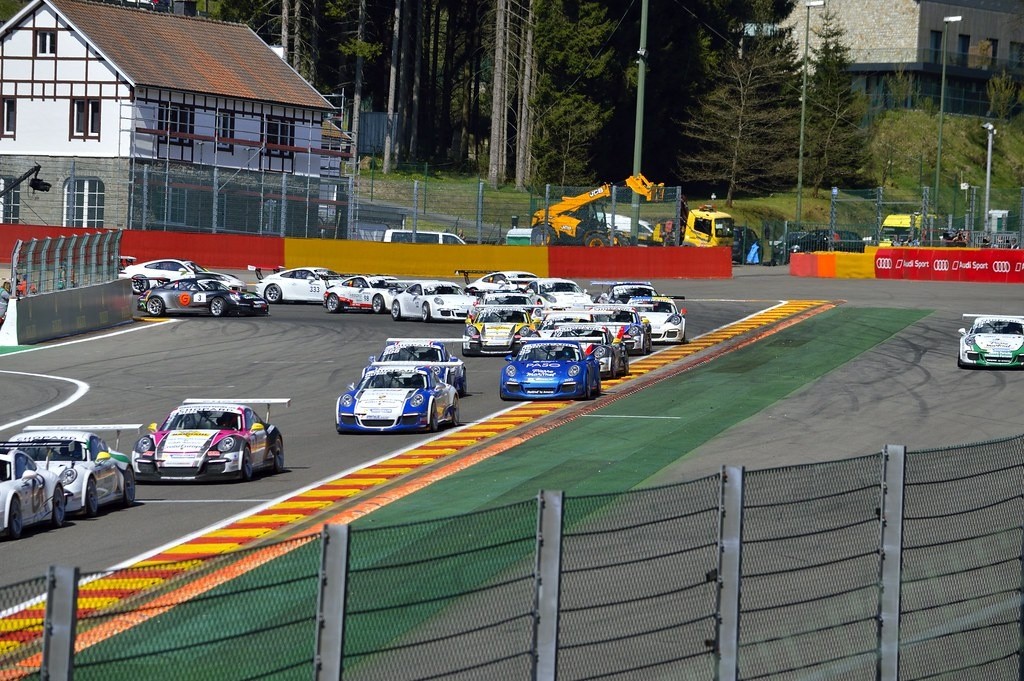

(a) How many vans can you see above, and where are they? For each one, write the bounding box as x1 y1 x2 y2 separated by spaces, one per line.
382 229 468 245
597 210 656 234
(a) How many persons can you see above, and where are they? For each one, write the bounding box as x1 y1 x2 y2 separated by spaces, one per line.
906 235 914 247
657 303 670 312
425 349 438 360
561 346 575 360
891 240 895 247
998 236 1003 243
458 229 466 242
825 233 834 251
410 375 424 387
1008 323 1023 334
983 236 991 248
512 311 524 322
1007 238 1020 250
943 228 969 243
218 413 239 430
620 311 632 322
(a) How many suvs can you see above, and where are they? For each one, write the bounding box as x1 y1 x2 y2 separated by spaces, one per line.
872 213 938 247
771 229 864 254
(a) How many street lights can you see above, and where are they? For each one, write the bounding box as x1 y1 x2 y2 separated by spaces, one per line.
934 15 963 214
981 123 994 234
796 1 827 227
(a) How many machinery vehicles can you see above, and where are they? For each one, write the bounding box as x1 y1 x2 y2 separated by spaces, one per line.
531 173 666 248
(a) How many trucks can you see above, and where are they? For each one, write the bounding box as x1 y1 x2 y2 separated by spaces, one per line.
623 204 736 252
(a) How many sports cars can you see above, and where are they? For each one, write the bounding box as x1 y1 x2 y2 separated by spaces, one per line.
132 397 290 482
455 269 687 400
132 278 271 317
334 336 471 433
385 279 481 322
958 314 1024 370
248 266 348 304
315 273 409 314
0 424 143 536
114 255 207 292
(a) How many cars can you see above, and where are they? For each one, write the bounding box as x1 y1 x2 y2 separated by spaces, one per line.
732 226 762 262
